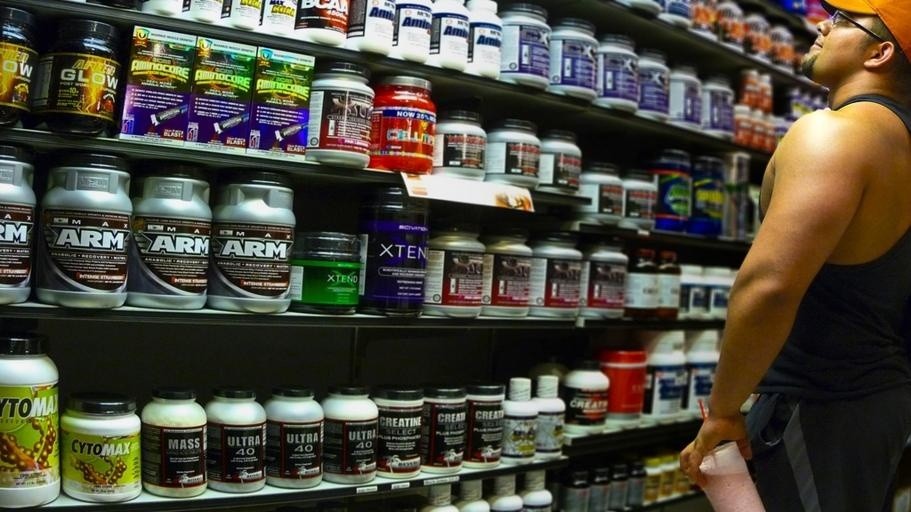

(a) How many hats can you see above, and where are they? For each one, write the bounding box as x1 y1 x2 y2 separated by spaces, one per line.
817 0 910 55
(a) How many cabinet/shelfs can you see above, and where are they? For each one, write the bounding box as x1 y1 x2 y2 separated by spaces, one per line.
0 0 834 512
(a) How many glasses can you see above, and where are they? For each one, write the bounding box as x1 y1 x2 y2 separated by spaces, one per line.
829 8 894 52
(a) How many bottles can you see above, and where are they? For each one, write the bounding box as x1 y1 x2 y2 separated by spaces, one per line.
0 0 833 512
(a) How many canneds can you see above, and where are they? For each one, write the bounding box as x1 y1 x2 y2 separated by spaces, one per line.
368 74 436 175
0 4 42 128
650 146 751 242
27 17 123 137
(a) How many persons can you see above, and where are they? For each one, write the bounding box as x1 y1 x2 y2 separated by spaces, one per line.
677 1 911 512
